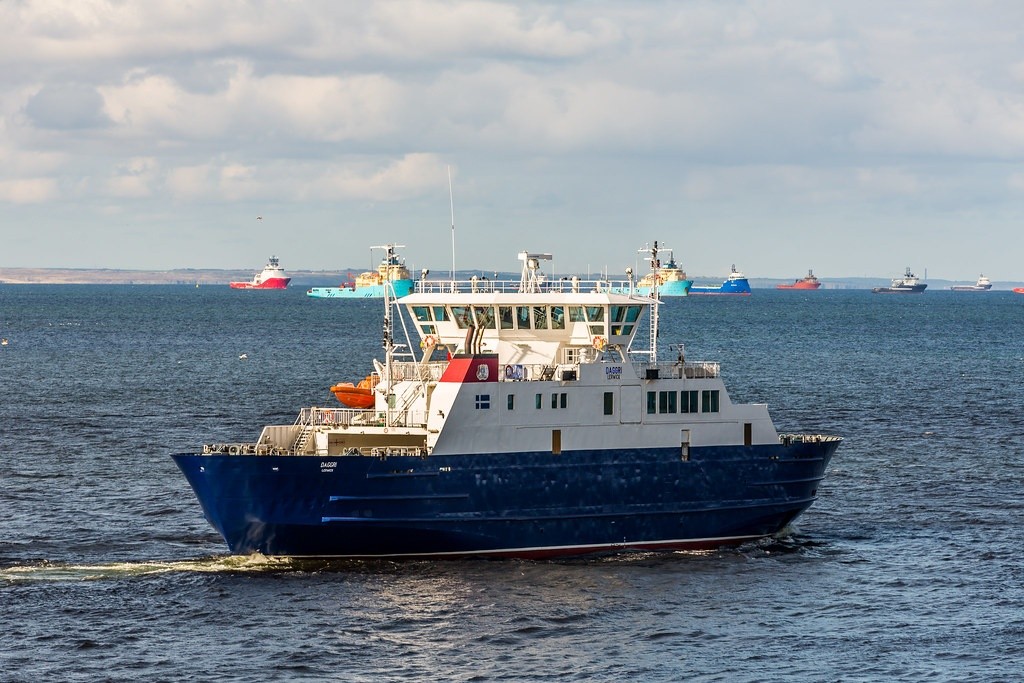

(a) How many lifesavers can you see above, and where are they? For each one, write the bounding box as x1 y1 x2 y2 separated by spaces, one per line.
593 336 602 348
426 336 435 346
506 365 513 377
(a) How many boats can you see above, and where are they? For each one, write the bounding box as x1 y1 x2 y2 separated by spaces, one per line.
329 387 375 409
776 268 822 290
950 273 993 292
599 242 694 297
168 163 847 562
871 266 928 294
688 264 752 298
307 243 418 300
230 255 292 290
